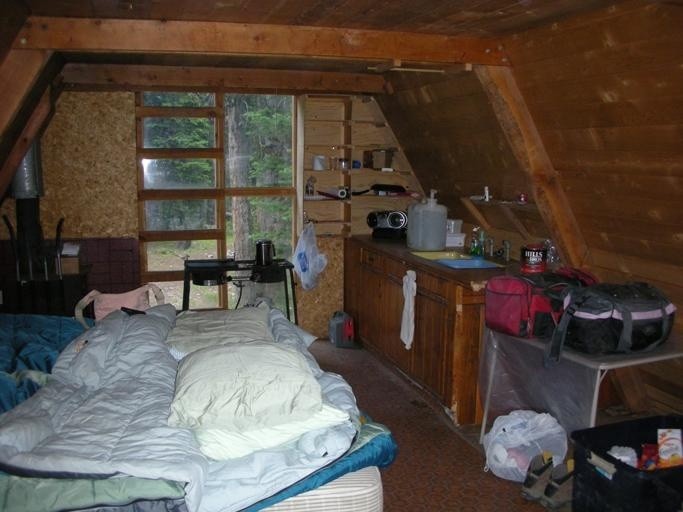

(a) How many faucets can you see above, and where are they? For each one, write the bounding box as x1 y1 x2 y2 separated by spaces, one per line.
502 240 511 262
53 242 82 277
488 238 495 256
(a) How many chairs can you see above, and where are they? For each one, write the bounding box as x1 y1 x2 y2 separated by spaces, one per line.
77 236 142 316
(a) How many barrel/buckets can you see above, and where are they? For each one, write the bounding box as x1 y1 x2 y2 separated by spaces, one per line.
327 310 355 349
406 198 448 252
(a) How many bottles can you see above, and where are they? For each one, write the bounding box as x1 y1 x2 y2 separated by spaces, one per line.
484 186 489 202
305 180 313 195
470 240 477 248
255 239 273 267
329 145 337 170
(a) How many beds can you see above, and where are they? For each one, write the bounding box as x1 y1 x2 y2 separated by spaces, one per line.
0 306 397 512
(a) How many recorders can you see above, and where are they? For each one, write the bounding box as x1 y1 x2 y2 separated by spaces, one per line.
367 210 408 229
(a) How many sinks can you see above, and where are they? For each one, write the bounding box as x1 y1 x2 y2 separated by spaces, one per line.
438 259 508 270
408 249 473 261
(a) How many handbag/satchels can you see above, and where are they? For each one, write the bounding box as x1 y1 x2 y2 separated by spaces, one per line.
74 282 167 332
544 278 678 369
483 265 599 339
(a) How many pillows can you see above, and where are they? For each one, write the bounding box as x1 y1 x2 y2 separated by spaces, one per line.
163 340 326 429
191 395 351 462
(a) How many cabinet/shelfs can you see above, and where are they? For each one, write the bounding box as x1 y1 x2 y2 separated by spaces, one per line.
342 228 552 432
299 91 425 229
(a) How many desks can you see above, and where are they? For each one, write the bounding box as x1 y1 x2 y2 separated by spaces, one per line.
475 318 681 455
180 257 299 327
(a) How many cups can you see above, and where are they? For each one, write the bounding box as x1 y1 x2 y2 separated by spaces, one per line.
520 243 547 273
352 160 360 168
518 191 528 202
447 219 462 233
488 239 494 257
311 155 324 171
338 159 348 169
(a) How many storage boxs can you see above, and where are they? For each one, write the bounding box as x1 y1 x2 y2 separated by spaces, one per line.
566 409 682 512
